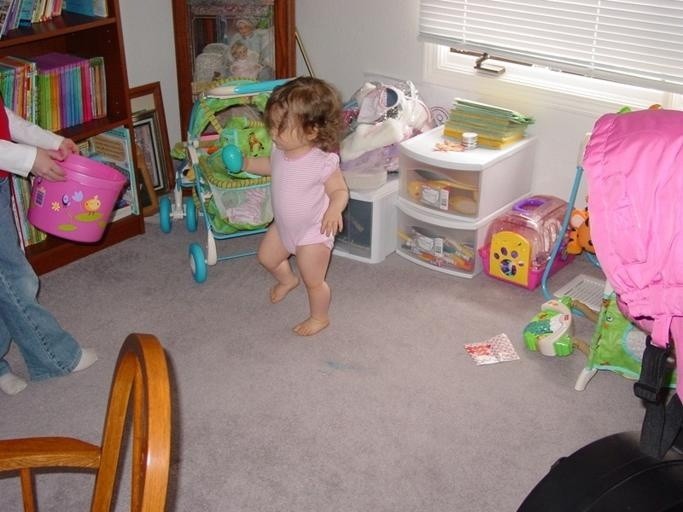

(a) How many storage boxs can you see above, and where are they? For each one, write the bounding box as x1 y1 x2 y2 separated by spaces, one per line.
332 174 397 264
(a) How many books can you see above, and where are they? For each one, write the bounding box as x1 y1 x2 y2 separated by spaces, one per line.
1 52 107 132
11 174 48 254
77 124 141 222
0 0 109 38
443 97 537 150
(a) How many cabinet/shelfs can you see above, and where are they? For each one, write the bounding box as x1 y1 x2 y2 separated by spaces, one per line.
395 124 536 280
0 1 145 276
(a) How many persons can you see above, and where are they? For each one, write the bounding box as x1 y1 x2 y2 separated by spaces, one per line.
226 76 350 335
225 16 275 80
0 91 99 395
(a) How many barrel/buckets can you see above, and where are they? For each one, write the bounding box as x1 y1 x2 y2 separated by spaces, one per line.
26 154 127 243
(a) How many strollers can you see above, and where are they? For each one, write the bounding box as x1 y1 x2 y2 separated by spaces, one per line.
156 78 301 284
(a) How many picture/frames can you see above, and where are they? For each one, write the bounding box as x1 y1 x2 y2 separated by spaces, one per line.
128 81 175 217
171 0 295 199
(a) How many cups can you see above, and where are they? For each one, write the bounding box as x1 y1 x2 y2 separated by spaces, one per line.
462 132 479 147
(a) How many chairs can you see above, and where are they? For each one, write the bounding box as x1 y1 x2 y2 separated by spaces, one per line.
0 332 172 512
